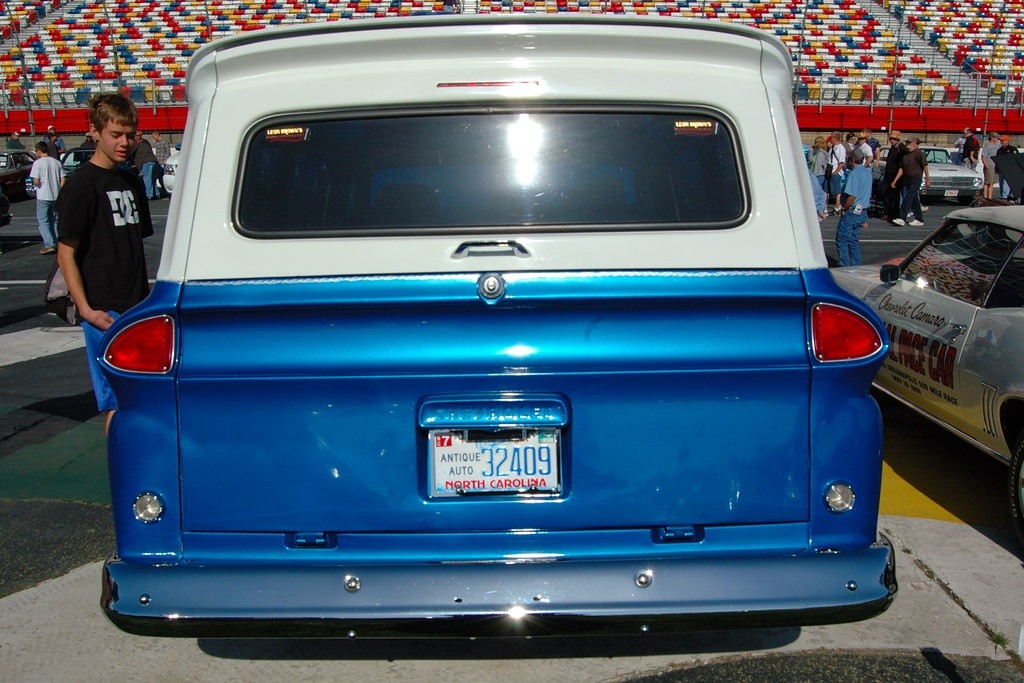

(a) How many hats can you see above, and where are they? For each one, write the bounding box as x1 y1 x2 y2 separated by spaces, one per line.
12 131 20 136
998 135 1009 141
48 126 54 130
991 132 1000 137
889 131 902 141
904 136 917 143
850 149 864 162
858 131 869 138
846 132 855 142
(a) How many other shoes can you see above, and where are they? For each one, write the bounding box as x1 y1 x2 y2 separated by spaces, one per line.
818 211 826 218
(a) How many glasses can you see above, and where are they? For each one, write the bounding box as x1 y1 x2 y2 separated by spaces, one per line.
858 137 866 140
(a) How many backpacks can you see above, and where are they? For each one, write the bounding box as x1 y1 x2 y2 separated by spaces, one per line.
824 163 833 181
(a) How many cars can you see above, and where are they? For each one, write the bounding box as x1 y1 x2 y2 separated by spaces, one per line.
828 206 1024 565
25 149 96 199
92 7 898 641
0 148 41 203
161 142 182 194
871 145 984 206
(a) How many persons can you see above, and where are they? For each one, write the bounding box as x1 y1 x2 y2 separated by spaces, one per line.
29 141 66 255
6 132 25 150
808 128 881 266
885 130 930 226
954 128 980 172
128 131 171 201
55 92 154 329
981 132 1002 199
43 125 65 161
80 133 96 150
995 135 1019 199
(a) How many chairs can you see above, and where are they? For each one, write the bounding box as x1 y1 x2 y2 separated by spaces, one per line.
0 0 1024 111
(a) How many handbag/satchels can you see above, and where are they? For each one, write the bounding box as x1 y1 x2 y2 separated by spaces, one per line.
842 163 846 171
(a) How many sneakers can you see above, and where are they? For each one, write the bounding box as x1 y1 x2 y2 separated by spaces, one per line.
828 207 841 216
909 219 924 225
893 219 905 226
40 247 56 255
